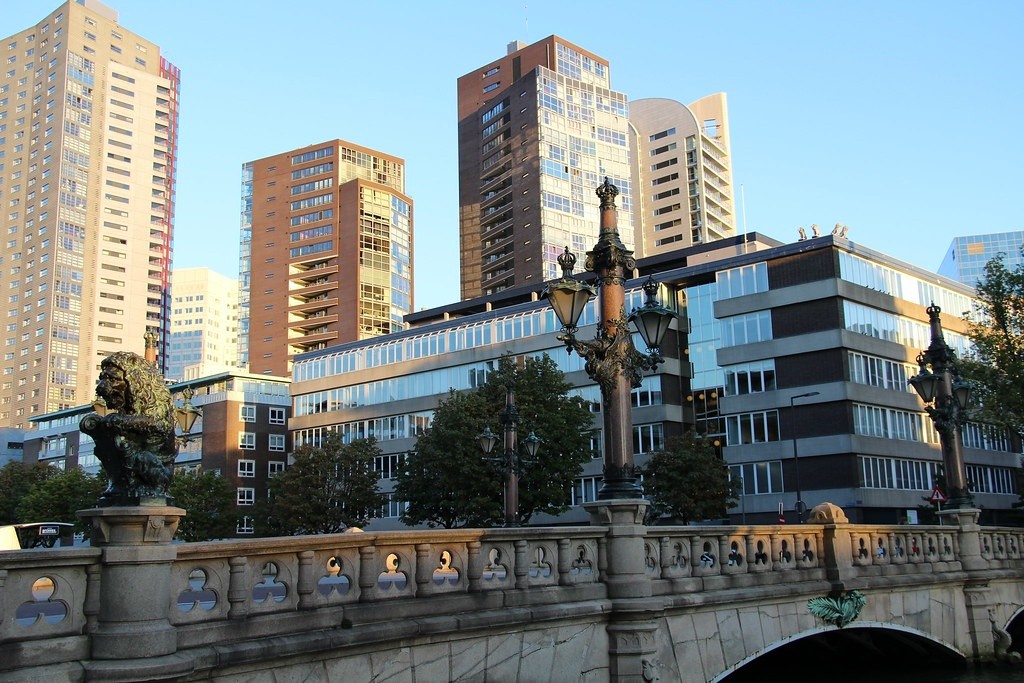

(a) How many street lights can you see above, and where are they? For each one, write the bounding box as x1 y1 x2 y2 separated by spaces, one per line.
540 173 682 499
477 385 545 527
904 300 981 509
790 392 820 525
